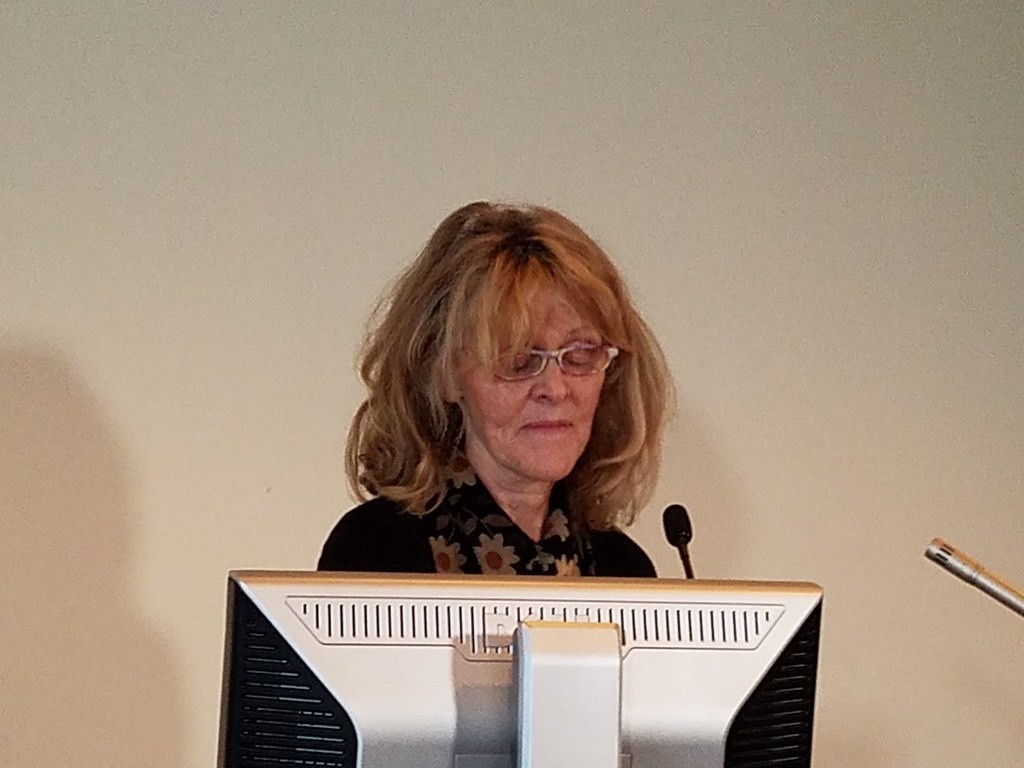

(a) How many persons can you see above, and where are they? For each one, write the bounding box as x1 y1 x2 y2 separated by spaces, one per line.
316 202 674 580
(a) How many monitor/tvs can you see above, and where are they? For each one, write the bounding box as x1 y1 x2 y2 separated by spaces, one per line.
216 569 822 767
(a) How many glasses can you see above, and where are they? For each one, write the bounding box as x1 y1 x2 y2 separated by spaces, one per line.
485 343 620 381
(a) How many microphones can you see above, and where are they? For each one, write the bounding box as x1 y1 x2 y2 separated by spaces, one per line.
662 504 694 580
923 536 1024 619
